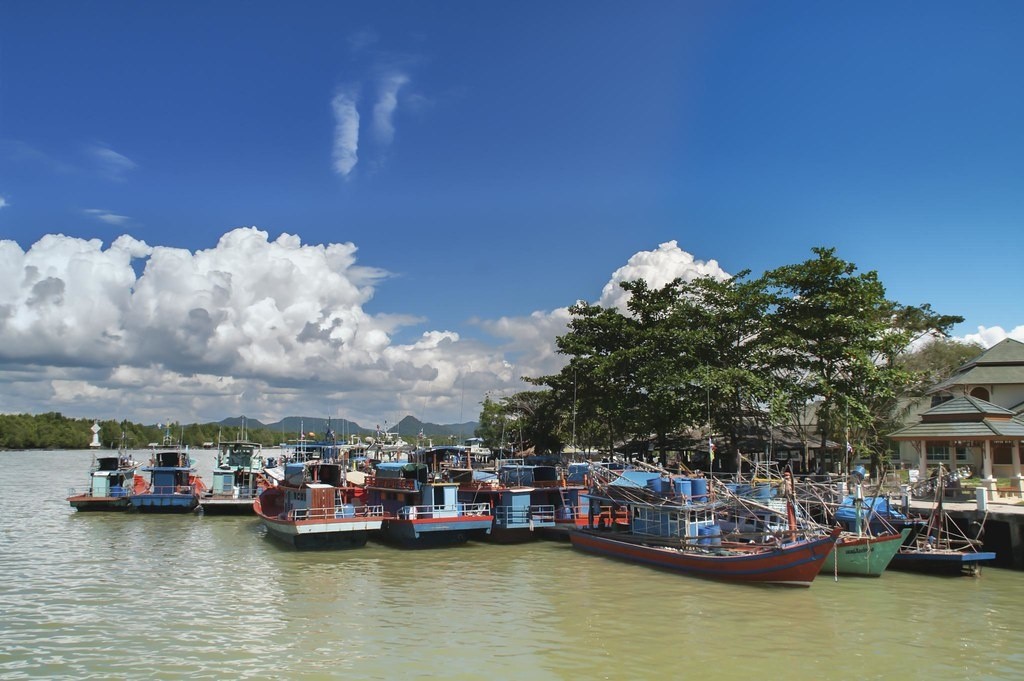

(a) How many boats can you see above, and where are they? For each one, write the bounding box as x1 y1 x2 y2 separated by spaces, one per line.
63 413 998 592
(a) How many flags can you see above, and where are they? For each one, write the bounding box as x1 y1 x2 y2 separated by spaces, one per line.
709 438 716 462
847 442 853 452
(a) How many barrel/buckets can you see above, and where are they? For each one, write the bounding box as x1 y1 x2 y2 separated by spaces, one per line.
852 466 865 479
120 488 128 497
559 505 571 519
726 483 778 499
698 525 721 551
110 486 121 497
645 476 708 505
